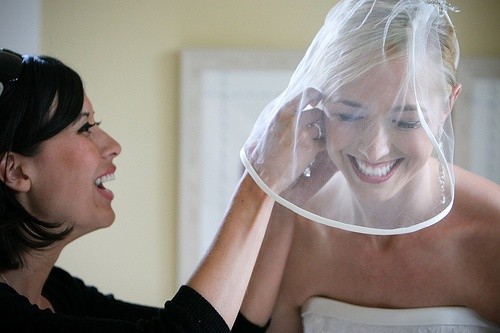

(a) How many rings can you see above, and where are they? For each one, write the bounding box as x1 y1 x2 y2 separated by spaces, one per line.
309 121 323 139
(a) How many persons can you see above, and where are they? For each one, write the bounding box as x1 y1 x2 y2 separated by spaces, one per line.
239 0 500 333
0 47 329 333
